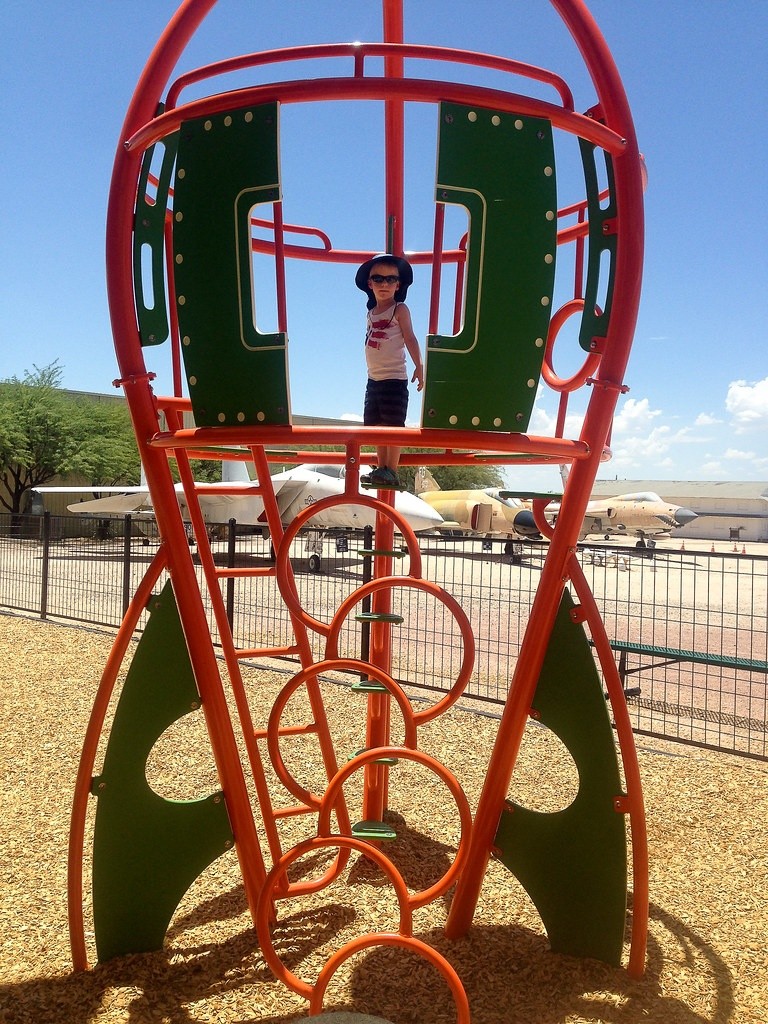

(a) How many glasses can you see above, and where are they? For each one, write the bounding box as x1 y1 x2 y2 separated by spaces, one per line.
369 274 399 284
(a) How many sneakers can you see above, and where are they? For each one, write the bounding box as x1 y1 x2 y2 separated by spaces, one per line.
371 466 399 486
360 465 377 483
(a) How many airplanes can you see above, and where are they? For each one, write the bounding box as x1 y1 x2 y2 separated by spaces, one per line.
30 446 447 575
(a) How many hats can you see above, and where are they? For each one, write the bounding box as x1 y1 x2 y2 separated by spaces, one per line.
355 254 414 298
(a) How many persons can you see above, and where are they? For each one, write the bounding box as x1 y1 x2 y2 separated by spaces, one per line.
355 254 424 486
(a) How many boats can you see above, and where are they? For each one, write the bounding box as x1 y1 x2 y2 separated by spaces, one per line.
411 464 701 565
